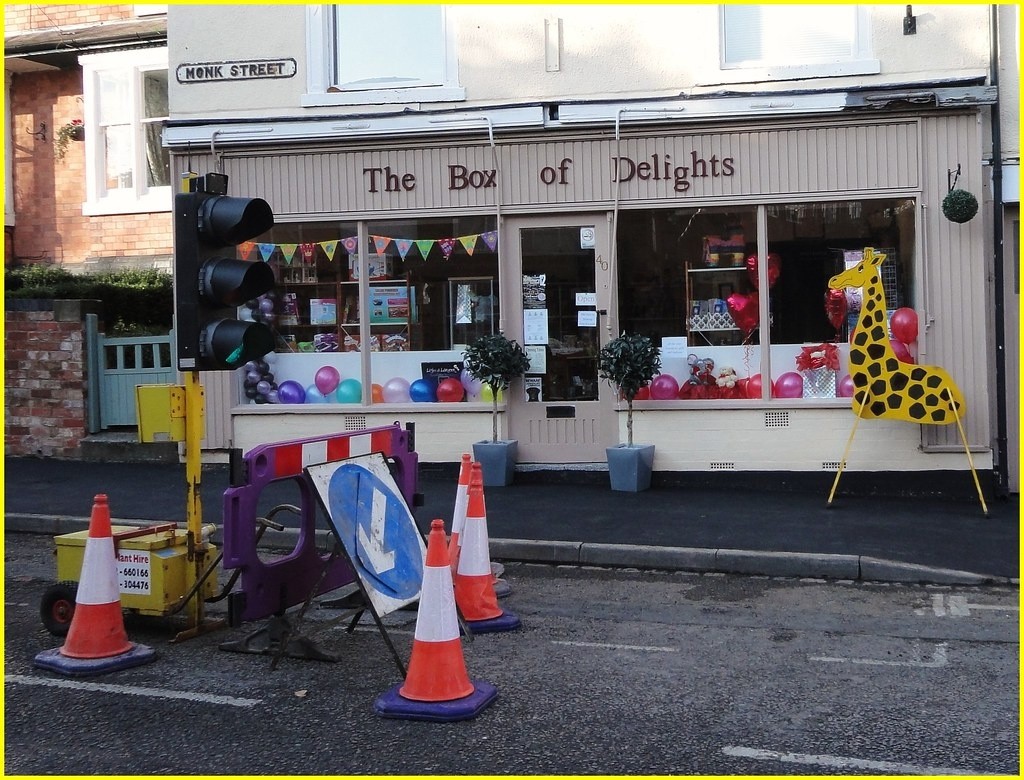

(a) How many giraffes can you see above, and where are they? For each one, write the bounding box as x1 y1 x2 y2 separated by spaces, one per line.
824 248 988 516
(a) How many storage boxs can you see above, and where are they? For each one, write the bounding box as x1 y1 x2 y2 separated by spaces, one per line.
276 292 300 326
313 333 339 353
371 287 416 322
690 299 728 315
297 342 313 353
382 334 408 352
276 334 295 352
309 297 338 326
348 253 394 281
343 335 382 352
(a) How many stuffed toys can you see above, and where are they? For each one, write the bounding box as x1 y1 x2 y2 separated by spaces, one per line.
715 366 738 388
687 354 715 385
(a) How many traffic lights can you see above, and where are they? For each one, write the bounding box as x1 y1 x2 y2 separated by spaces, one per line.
170 189 280 375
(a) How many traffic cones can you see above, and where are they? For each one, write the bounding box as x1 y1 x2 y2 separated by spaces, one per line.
370 516 500 722
446 452 505 580
30 493 159 676
449 480 523 633
449 462 512 598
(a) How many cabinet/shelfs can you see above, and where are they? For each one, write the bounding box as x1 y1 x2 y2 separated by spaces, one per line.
270 251 411 352
684 263 759 345
448 276 498 348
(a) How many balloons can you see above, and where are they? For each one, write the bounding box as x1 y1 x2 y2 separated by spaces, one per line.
623 386 649 401
649 375 679 400
238 293 502 404
840 374 854 399
774 372 804 399
826 288 847 331
748 373 774 398
888 306 919 364
727 253 780 334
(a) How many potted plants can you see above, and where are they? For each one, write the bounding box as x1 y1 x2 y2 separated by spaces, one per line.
462 335 530 486
596 332 661 494
